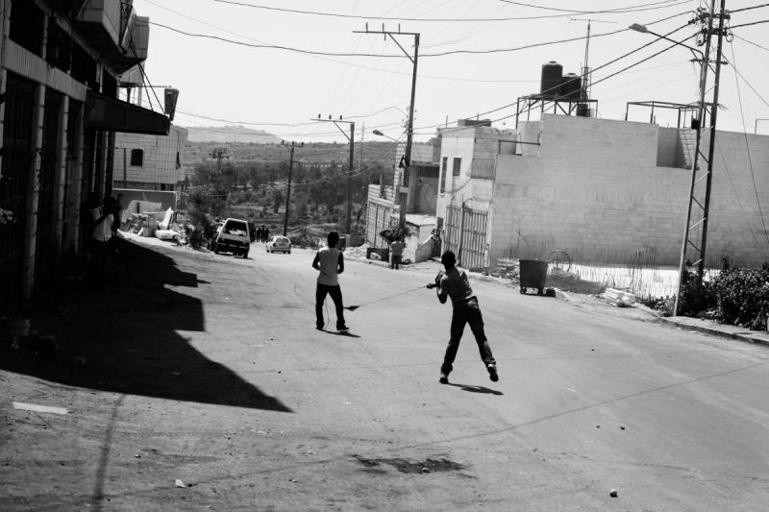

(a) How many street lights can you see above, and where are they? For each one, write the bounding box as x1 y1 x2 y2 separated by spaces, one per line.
625 24 727 316
372 128 411 231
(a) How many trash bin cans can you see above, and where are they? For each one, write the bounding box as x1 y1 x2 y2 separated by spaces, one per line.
338 236 346 253
518 259 548 295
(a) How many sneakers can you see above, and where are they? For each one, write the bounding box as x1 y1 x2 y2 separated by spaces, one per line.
487 363 499 383
336 324 349 330
440 373 449 384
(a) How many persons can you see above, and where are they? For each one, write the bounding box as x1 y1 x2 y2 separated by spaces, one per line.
312 232 349 331
88 197 117 292
111 193 123 236
391 237 404 269
427 250 498 383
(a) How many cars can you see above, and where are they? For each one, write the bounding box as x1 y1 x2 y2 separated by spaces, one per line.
211 216 250 258
265 234 291 255
